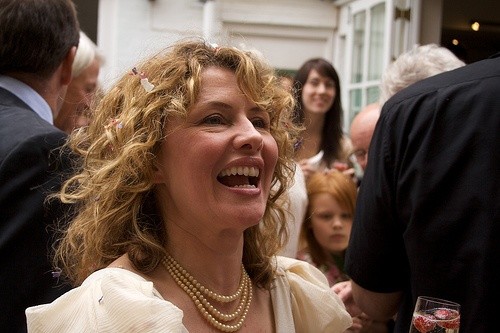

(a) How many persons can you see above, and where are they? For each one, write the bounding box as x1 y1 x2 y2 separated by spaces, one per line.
288 57 366 192
25 37 354 333
0 0 83 333
344 56 500 333
348 102 385 173
294 167 387 333
50 30 105 131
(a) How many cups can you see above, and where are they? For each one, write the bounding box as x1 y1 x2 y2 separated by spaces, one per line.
409 296 461 333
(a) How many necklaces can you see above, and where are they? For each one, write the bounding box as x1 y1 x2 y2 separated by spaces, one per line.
160 249 255 333
